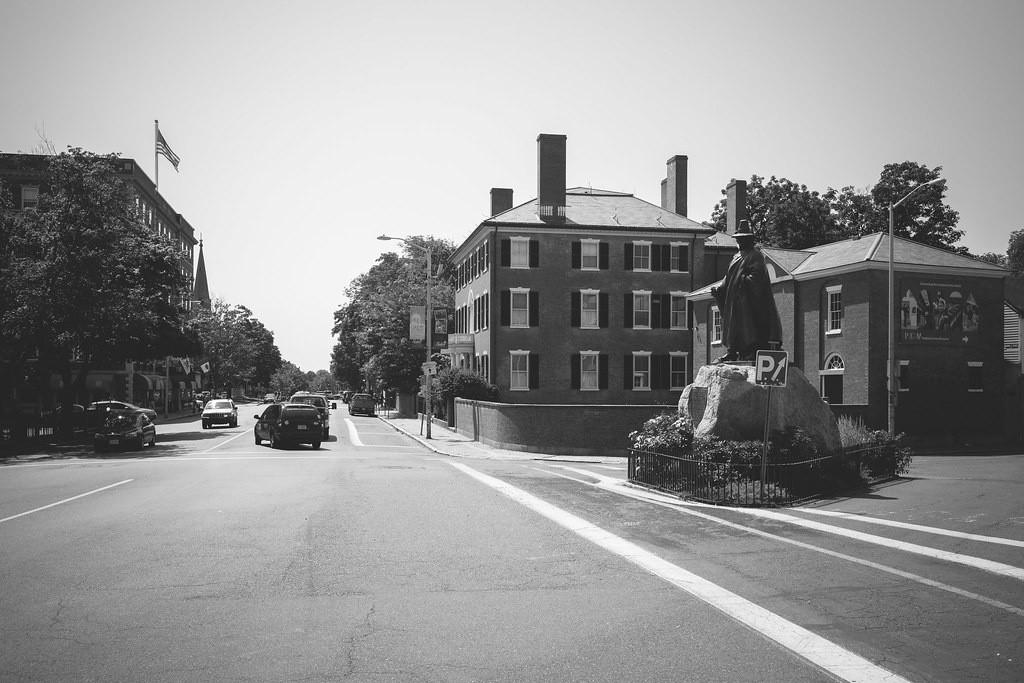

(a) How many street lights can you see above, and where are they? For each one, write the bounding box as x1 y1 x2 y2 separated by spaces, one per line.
884 176 946 438
375 234 434 442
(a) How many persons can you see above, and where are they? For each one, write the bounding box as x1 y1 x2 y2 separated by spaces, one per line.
903 290 979 331
711 220 783 363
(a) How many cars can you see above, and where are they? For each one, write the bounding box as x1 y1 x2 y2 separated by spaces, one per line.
85 400 157 426
264 393 277 404
348 393 376 416
91 408 157 452
285 389 338 440
338 389 356 405
200 397 241 429
253 402 324 450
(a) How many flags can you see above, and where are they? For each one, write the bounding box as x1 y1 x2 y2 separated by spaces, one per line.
180 357 190 375
157 130 179 172
201 362 210 373
